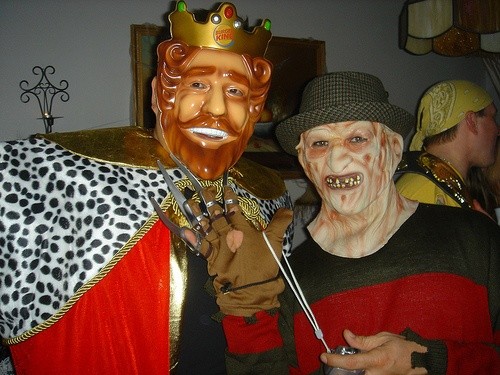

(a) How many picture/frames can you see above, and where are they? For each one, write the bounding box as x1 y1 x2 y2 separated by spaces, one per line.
130 23 328 182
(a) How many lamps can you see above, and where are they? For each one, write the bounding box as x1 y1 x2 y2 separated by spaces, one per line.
397 0 500 57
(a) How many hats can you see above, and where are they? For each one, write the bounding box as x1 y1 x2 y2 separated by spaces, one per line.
276 71 414 157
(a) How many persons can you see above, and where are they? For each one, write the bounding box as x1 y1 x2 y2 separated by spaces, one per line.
463 138 500 224
178 71 500 375
0 0 295 375
392 78 500 211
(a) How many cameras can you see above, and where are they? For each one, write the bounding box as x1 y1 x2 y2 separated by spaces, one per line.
322 346 366 375
